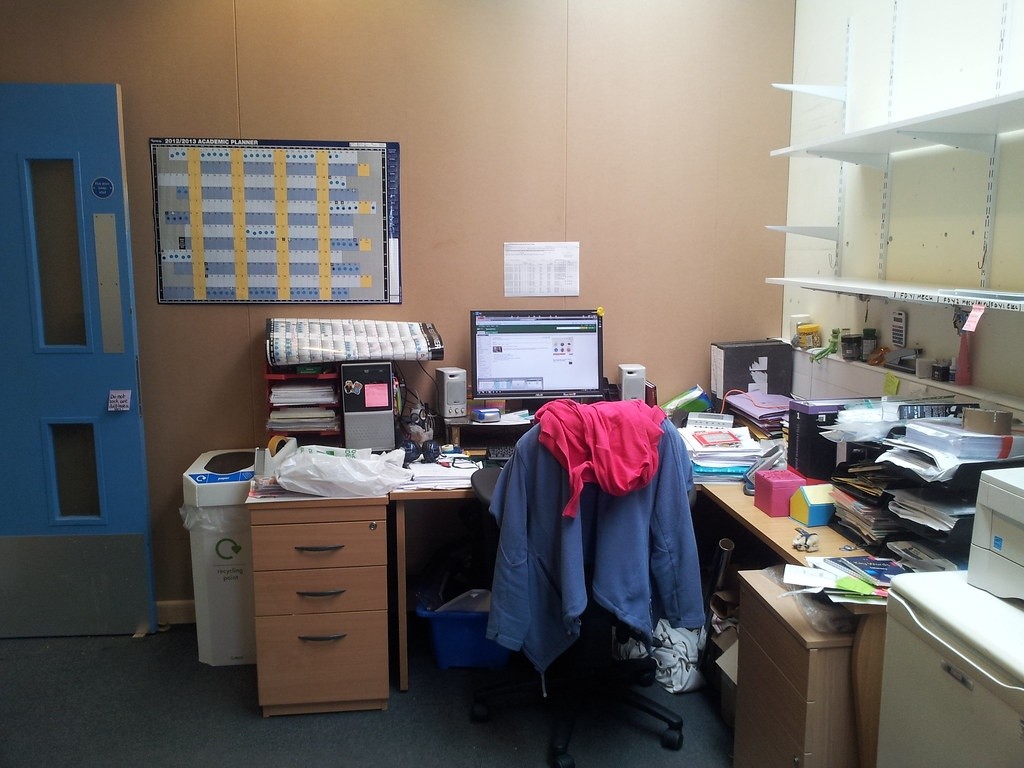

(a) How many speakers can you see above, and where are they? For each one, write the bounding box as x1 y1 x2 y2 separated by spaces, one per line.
435 367 467 418
617 364 646 404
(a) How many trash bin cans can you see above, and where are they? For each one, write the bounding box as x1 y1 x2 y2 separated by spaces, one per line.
182 448 259 666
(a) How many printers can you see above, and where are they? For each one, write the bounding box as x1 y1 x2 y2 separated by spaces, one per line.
966 467 1024 601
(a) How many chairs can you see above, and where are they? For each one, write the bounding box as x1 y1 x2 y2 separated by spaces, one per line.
468 397 708 768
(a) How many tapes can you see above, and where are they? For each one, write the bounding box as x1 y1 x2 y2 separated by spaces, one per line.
267 435 288 458
963 408 1013 434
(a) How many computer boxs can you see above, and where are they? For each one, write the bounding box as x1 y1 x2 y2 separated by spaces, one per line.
340 362 395 451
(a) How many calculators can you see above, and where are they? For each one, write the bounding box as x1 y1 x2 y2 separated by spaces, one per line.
891 311 907 347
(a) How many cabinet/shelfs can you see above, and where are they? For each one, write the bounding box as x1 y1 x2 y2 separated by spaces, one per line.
764 89 1024 311
245 489 391 720
730 566 860 768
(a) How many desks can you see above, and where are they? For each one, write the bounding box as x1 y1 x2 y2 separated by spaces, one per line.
389 479 702 692
704 483 887 768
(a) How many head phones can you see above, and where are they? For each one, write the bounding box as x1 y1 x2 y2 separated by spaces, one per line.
399 439 440 462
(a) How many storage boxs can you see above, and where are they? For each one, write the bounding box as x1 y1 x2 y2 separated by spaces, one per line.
753 469 808 517
417 577 514 670
961 407 1014 436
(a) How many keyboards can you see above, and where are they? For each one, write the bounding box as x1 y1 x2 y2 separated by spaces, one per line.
486 445 515 459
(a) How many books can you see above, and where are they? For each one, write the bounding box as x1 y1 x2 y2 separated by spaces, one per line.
726 386 790 453
269 386 337 431
825 554 913 587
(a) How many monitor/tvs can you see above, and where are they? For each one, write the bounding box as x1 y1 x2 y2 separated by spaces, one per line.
470 309 603 416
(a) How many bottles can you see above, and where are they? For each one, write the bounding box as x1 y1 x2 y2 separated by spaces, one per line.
862 328 877 360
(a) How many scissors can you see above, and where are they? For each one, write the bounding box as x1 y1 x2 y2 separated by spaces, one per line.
411 409 427 429
